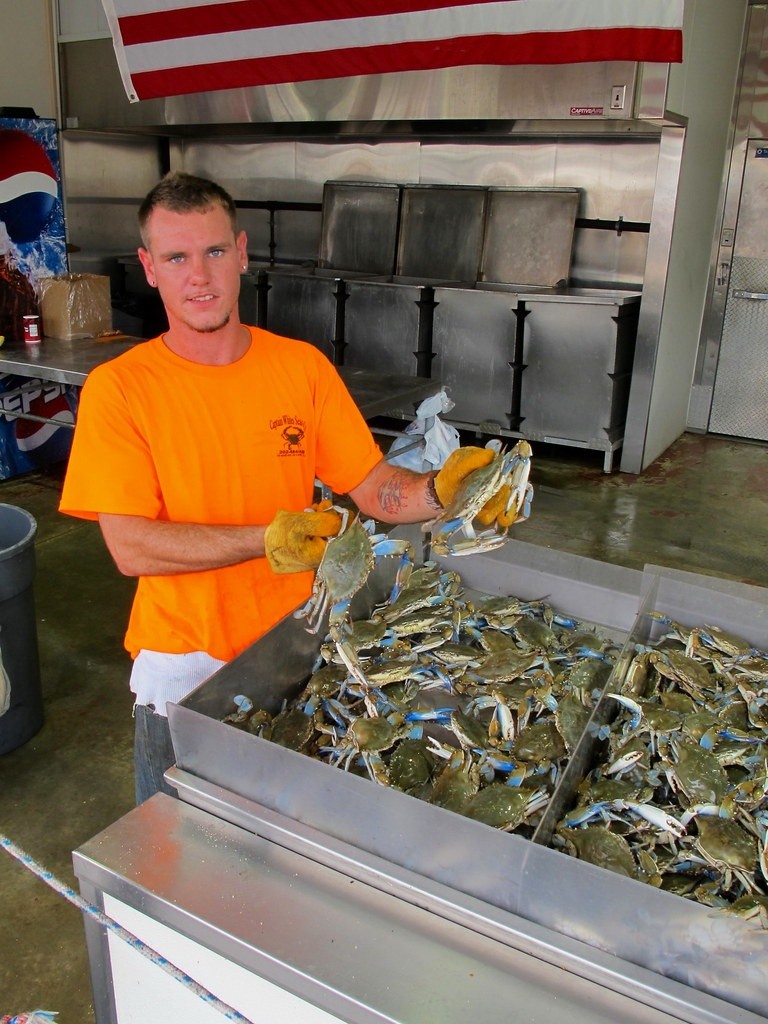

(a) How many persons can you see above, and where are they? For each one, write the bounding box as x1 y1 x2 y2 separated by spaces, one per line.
59 171 534 811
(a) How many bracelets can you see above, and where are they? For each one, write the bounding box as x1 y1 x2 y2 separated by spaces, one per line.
427 469 444 509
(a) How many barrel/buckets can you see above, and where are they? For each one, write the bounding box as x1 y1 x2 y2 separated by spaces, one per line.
0 503 44 756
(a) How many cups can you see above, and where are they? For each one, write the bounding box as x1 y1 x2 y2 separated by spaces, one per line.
23 316 41 344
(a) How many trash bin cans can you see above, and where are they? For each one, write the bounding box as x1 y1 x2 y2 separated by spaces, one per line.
0 502 48 759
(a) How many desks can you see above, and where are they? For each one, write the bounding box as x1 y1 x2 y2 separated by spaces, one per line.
0 335 442 507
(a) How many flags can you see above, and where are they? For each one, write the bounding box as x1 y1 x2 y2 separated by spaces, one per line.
102 0 683 103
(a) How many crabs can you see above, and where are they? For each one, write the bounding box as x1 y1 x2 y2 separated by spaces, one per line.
549 610 768 933
292 504 416 688
219 559 626 833
431 439 535 558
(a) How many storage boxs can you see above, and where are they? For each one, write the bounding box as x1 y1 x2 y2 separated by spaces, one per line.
37 271 114 341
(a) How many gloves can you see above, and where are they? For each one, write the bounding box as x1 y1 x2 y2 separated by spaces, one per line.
435 446 516 526
265 500 351 571
